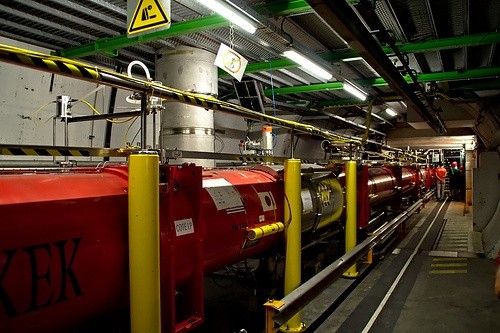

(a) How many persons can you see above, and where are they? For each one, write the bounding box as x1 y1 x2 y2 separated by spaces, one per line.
449 162 459 201
455 165 465 201
435 165 442 199
436 163 447 200
